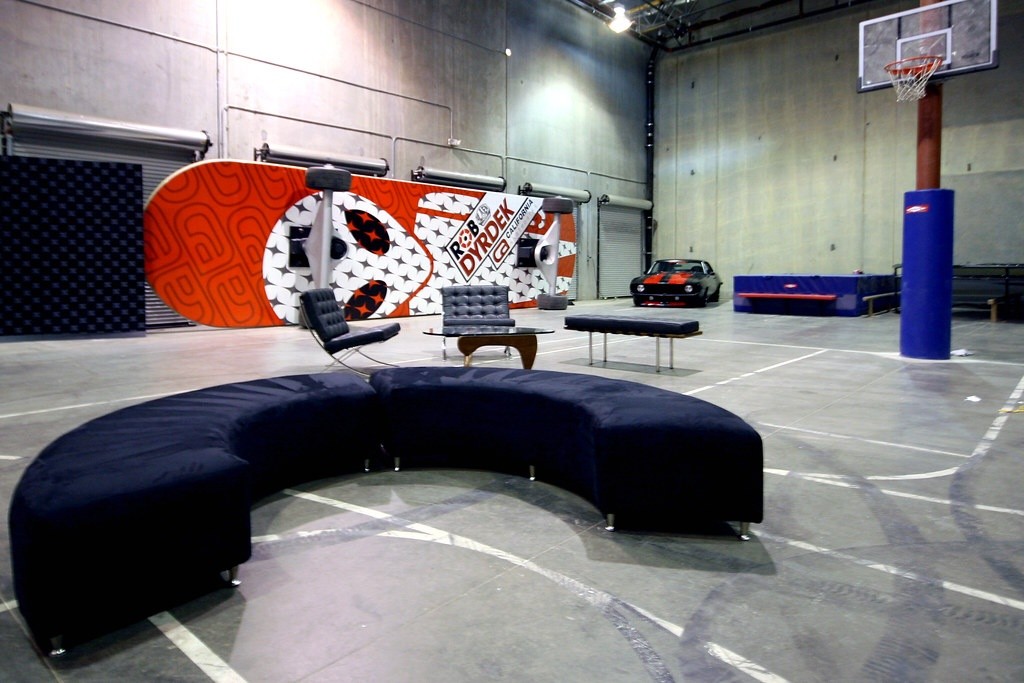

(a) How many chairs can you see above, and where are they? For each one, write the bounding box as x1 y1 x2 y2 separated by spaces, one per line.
441 285 514 359
301 288 400 376
691 266 703 272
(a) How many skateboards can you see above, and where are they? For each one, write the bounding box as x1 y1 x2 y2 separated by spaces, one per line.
144 156 577 315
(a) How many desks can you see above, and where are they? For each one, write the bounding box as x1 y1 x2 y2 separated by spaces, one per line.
421 324 556 370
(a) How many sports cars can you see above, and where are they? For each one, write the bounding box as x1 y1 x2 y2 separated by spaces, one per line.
629 259 723 308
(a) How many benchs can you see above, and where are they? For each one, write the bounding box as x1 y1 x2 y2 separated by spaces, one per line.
12 367 763 662
739 293 836 315
565 314 703 374
